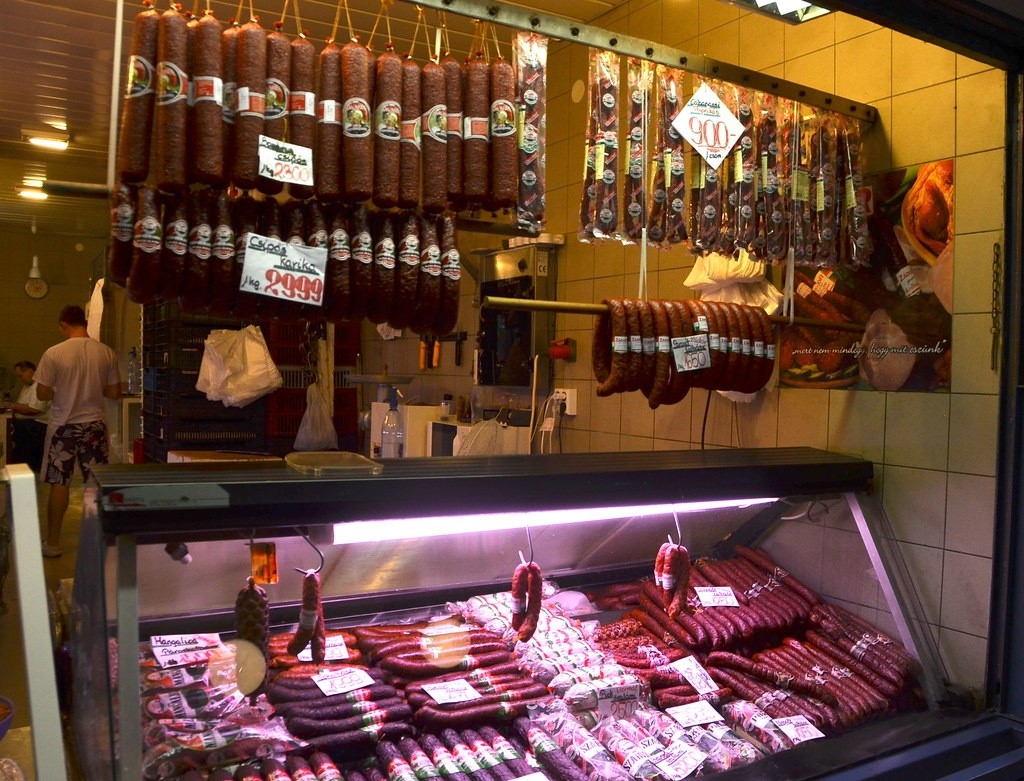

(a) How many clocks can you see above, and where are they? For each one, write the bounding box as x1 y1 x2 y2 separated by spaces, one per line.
25 278 48 299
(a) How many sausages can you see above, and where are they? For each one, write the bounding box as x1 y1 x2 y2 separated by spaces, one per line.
105 7 919 781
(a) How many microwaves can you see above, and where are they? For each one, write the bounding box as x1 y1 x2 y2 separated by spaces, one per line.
425 420 531 458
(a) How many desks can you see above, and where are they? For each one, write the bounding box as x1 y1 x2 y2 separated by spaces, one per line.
117 395 142 464
0 410 12 469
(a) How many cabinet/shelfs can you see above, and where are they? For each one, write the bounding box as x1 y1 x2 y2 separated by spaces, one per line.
369 401 443 458
426 420 531 459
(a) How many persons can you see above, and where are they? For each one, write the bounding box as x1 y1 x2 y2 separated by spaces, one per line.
31 303 122 557
1 361 52 471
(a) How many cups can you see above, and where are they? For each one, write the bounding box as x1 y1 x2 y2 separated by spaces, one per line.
378 383 406 459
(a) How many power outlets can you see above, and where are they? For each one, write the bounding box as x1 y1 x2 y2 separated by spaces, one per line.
553 387 577 416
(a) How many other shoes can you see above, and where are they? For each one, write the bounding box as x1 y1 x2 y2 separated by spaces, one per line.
41 536 63 558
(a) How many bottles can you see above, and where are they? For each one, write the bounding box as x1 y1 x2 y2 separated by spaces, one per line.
439 393 457 422
126 346 141 395
